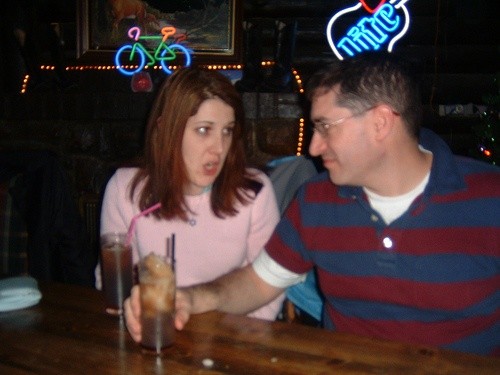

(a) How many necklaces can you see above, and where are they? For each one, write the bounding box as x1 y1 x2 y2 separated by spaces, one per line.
189 186 204 227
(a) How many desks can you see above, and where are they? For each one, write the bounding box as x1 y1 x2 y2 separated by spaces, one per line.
0 277 499 374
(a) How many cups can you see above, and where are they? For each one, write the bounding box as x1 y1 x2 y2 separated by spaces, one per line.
99 232 131 310
139 255 176 356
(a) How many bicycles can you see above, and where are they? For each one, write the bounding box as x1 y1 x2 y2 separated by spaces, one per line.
114 25 192 77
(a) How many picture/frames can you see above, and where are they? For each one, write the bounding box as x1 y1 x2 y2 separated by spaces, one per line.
75 0 243 62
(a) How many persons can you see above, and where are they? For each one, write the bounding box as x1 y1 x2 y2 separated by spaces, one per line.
123 48 500 356
0 145 97 289
94 65 284 324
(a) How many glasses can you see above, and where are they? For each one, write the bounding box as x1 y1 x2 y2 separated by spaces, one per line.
311 105 378 134
